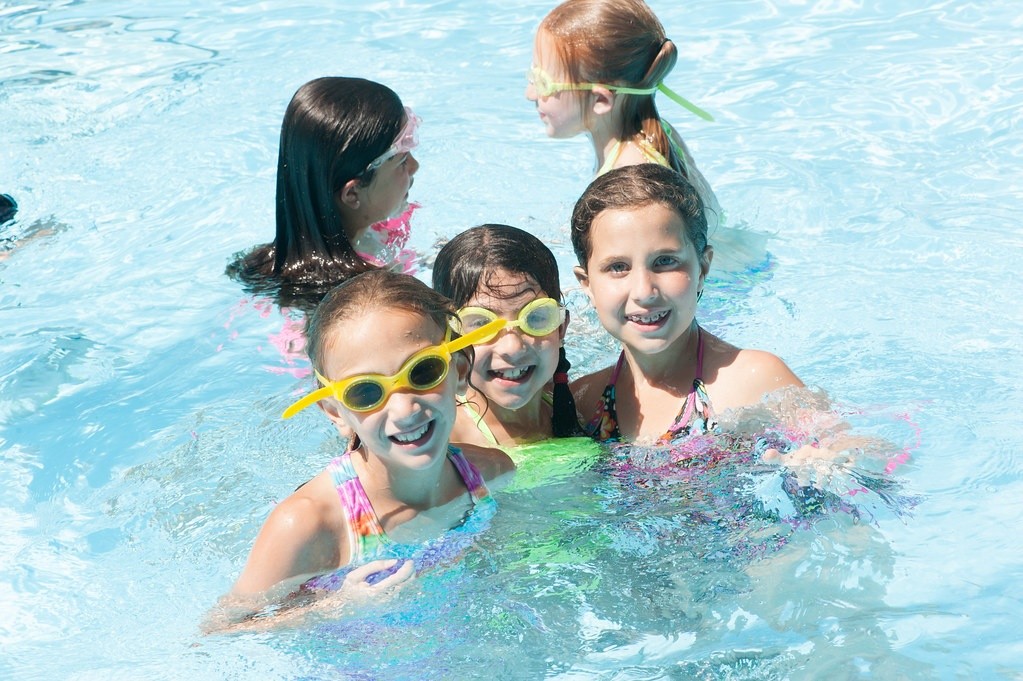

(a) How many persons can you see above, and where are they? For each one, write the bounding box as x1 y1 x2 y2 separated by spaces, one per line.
526 0 783 294
226 77 418 375
0 192 53 266
200 164 903 642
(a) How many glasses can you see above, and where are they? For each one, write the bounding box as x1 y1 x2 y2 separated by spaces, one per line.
448 298 566 344
281 316 511 422
528 63 715 121
356 106 422 177
